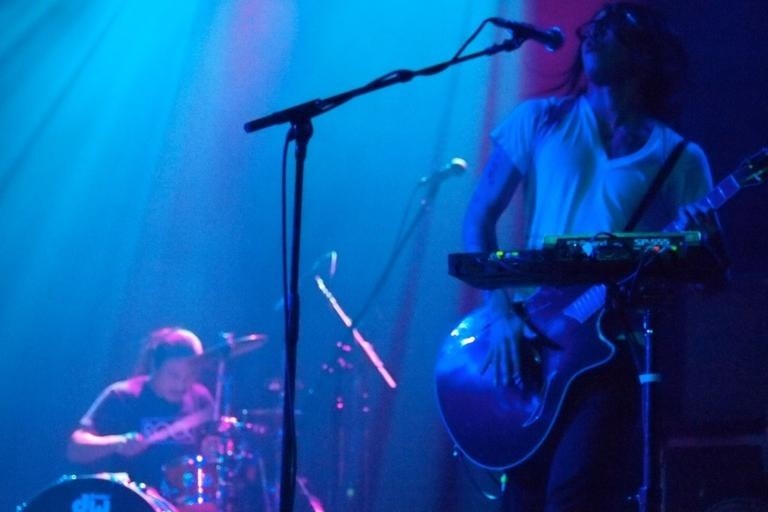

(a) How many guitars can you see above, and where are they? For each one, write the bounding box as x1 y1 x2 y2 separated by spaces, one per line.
436 153 763 472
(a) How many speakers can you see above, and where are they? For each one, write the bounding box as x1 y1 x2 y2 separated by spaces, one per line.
658 434 768 512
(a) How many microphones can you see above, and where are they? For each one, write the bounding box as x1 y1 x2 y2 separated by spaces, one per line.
421 157 469 187
491 17 567 53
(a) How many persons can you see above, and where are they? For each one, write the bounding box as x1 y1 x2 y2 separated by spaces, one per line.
461 4 716 509
66 327 214 484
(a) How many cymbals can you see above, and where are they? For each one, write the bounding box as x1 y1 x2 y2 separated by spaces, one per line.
188 334 264 361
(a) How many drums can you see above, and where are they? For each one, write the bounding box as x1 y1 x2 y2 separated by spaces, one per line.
15 472 177 512
163 455 252 510
